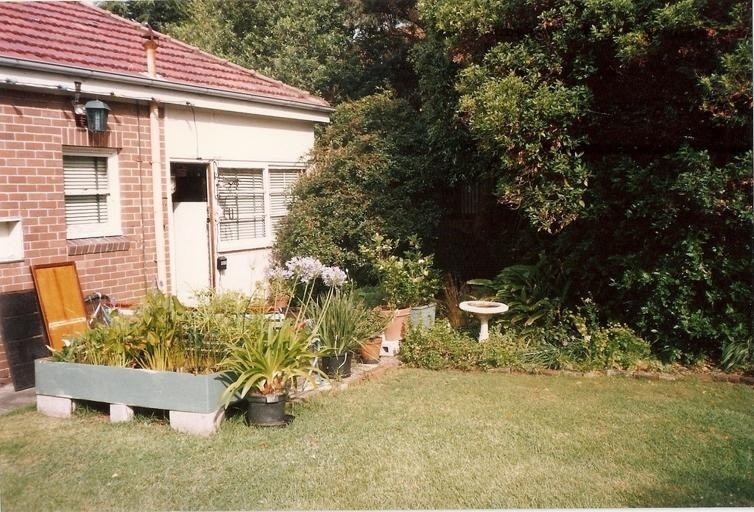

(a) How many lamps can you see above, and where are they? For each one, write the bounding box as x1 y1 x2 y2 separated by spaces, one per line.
74 81 111 134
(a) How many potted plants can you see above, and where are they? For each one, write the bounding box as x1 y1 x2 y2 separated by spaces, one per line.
297 232 437 379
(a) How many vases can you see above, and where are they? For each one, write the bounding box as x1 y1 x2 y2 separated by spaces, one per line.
245 389 288 426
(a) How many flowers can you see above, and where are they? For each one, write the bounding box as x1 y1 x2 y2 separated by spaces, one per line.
215 247 340 411
